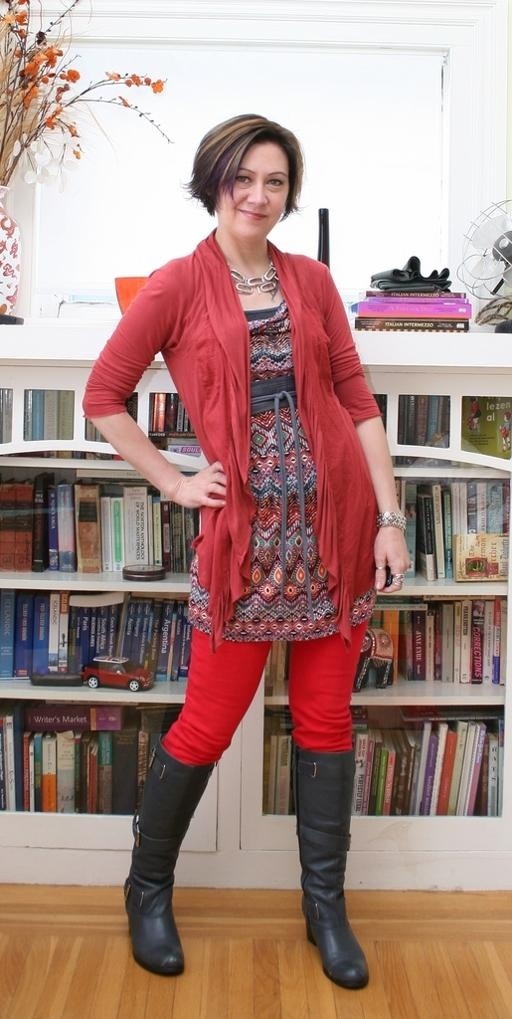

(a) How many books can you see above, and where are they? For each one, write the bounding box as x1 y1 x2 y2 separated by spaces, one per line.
343 286 473 332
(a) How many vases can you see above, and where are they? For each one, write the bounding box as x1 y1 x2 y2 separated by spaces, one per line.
0 185 22 325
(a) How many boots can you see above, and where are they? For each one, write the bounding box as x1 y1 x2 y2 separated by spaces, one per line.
123 733 216 977
293 745 369 990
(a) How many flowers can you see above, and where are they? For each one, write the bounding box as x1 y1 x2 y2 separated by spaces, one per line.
1 0 173 187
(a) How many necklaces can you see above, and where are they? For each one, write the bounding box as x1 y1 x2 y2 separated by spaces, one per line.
224 259 280 294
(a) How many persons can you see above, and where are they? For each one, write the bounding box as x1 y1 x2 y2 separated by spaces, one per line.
82 112 416 989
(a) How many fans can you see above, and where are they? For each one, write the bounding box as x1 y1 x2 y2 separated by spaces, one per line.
453 198 512 333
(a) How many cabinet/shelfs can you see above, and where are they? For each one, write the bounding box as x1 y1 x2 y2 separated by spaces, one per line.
0 328 512 889
